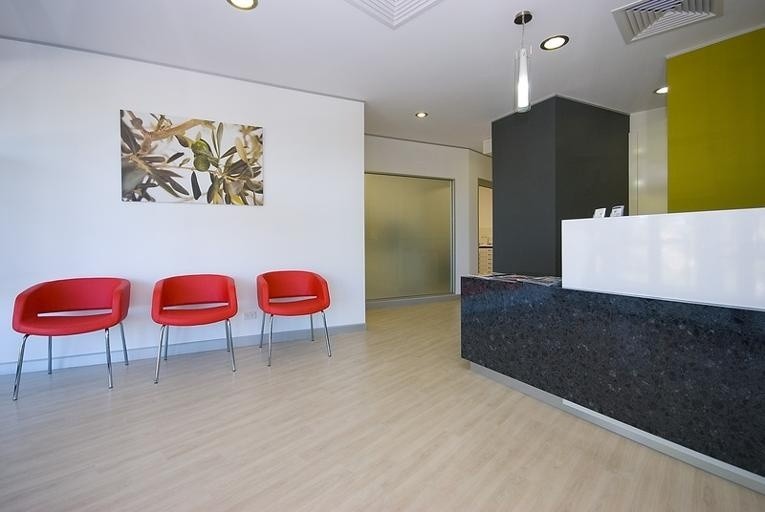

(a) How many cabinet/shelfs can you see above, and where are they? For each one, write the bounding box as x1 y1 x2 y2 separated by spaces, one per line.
478 244 494 276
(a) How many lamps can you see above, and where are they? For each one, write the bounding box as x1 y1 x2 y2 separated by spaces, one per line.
513 11 535 115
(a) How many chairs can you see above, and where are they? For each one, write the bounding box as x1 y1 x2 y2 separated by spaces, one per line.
256 270 332 366
11 277 131 402
150 274 238 384
